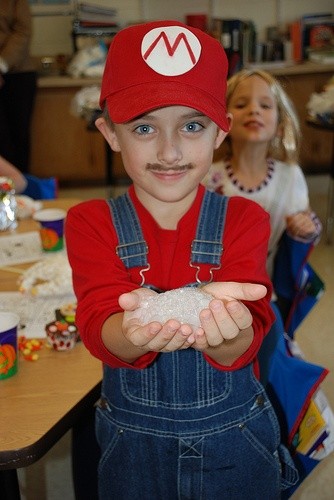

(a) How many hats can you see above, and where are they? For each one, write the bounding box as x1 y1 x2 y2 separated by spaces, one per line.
100 20 235 134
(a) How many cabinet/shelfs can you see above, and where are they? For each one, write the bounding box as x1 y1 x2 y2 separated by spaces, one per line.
30 93 109 182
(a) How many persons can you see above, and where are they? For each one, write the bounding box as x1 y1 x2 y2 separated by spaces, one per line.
200 70 321 341
0 0 37 174
64 20 300 500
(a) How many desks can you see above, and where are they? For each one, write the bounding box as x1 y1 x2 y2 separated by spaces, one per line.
0 197 105 500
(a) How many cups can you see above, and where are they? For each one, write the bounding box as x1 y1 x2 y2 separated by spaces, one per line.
0 310 21 381
33 208 66 252
